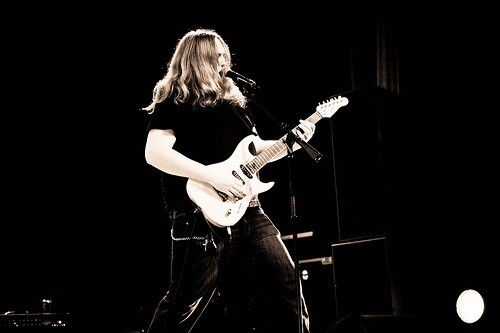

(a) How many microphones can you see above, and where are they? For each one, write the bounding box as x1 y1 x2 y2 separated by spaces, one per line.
220 68 256 88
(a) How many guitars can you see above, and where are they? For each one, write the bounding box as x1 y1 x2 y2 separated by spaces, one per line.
186 95 348 228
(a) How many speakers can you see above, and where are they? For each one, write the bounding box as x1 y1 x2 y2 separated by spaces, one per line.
296 257 340 333
330 236 400 316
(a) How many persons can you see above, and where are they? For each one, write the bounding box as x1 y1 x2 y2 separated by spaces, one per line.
144 28 315 333
